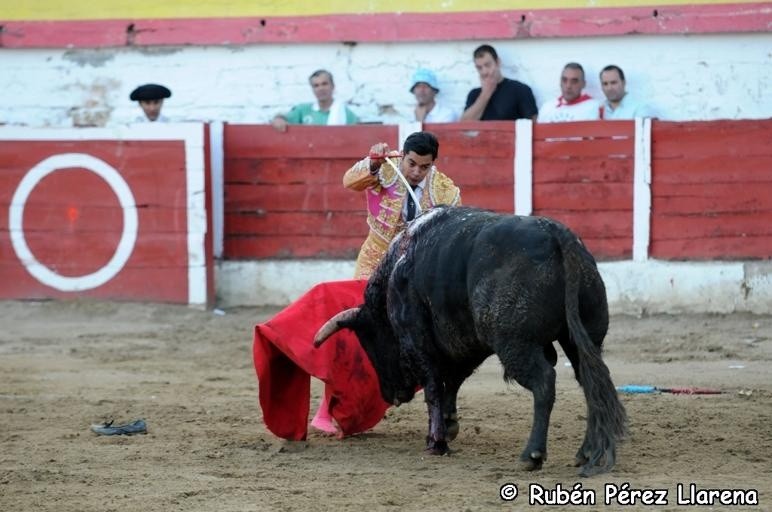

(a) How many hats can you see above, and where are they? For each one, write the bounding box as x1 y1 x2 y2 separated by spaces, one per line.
130 84 173 101
408 67 441 95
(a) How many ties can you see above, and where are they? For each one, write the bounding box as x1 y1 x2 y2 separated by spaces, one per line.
406 184 417 221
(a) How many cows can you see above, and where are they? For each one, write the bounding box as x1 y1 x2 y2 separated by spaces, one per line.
311 202 630 479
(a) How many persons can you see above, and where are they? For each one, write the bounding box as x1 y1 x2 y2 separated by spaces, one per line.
536 62 604 125
266 68 364 134
306 130 464 436
400 67 459 126
457 44 539 122
597 65 660 120
126 83 174 124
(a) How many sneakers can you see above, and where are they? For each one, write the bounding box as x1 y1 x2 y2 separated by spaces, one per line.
92 418 147 435
